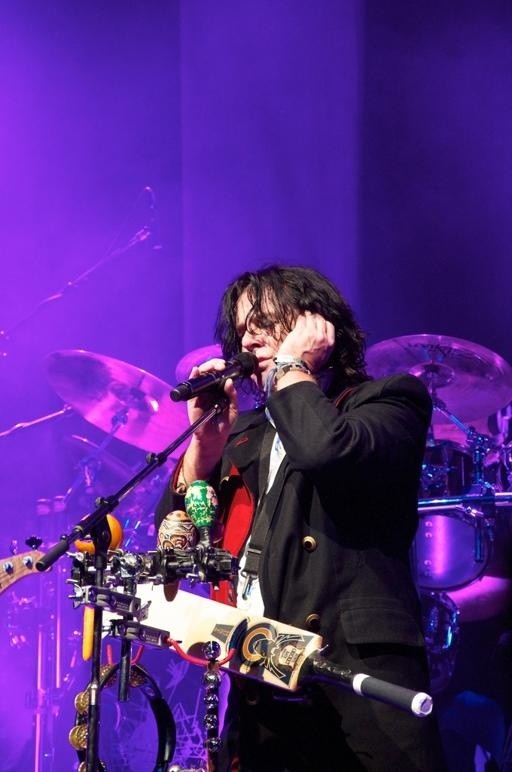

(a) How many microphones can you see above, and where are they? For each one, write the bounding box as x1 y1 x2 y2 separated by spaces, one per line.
168 350 258 403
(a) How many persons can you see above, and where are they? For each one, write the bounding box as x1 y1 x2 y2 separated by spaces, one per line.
425 436 511 771
152 262 449 771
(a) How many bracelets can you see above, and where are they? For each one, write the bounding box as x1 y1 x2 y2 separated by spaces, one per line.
262 359 313 392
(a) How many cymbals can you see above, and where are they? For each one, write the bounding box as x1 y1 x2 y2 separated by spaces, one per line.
175 344 266 412
42 348 191 459
65 431 134 476
364 333 512 424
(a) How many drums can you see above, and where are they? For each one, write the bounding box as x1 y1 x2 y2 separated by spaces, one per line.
409 439 493 590
